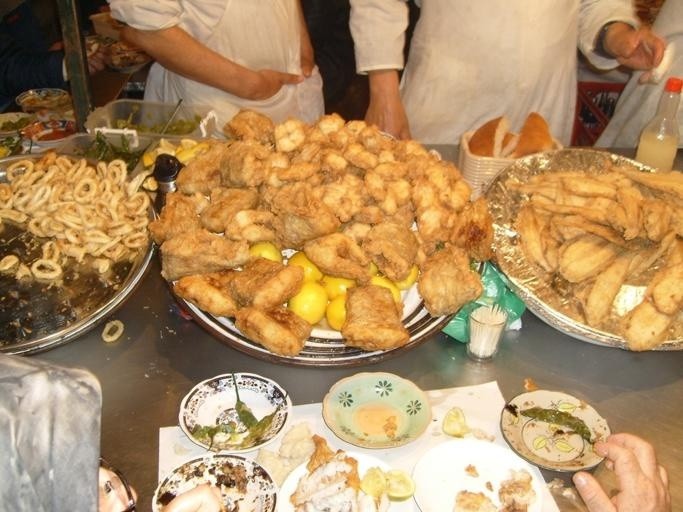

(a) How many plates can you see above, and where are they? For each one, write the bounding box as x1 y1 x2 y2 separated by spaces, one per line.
178 372 294 454
160 165 486 369
499 389 613 474
486 147 683 353
0 153 161 360
150 455 278 510
325 372 432 450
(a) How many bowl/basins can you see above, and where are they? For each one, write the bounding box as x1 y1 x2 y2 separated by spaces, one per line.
14 87 70 110
459 131 561 193
88 13 118 39
109 52 150 73
82 99 219 142
26 119 77 148
38 126 156 178
0 132 34 159
0 112 39 135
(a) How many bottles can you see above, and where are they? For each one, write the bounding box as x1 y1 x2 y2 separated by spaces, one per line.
633 77 682 171
154 152 183 197
604 99 614 119
578 91 591 119
598 91 609 112
583 97 597 127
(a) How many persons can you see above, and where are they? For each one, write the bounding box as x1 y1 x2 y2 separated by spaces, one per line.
595 0 683 148
1 353 674 512
109 0 324 127
351 0 663 140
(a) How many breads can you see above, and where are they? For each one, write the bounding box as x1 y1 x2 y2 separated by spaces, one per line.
502 129 520 157
510 112 552 159
469 117 508 158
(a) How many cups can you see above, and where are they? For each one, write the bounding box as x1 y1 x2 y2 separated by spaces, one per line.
467 301 508 363
